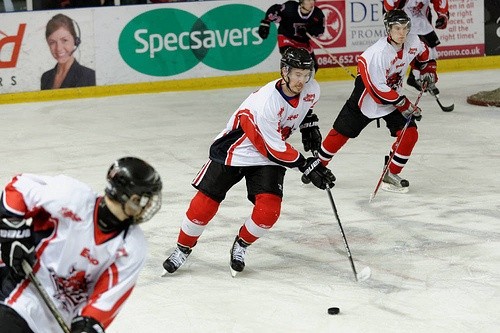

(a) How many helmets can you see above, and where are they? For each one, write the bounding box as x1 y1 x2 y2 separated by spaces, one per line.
280 47 312 70
105 157 163 204
383 10 411 25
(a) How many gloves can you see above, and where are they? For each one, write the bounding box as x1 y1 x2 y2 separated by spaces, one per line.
258 21 270 39
435 11 449 30
300 157 336 190
299 114 322 157
0 217 37 279
394 95 422 121
70 315 104 333
414 60 438 90
297 28 313 38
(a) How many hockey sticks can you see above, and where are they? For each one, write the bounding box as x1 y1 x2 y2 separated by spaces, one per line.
310 146 370 282
306 31 357 79
428 82 455 112
369 77 429 203
22 259 71 333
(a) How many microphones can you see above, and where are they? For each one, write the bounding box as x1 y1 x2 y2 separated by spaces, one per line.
70 45 79 56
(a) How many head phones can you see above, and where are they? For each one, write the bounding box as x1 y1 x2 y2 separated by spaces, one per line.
72 20 80 45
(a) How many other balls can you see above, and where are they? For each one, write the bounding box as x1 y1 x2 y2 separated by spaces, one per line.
327 307 339 315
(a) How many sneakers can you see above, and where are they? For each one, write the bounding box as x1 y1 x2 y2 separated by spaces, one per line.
405 68 440 98
301 174 311 186
229 226 251 278
161 240 197 276
382 156 409 193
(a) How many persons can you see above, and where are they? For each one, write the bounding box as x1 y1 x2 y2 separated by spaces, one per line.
301 10 438 191
41 13 95 89
158 46 337 276
0 157 162 333
258 0 325 73
384 0 450 97
